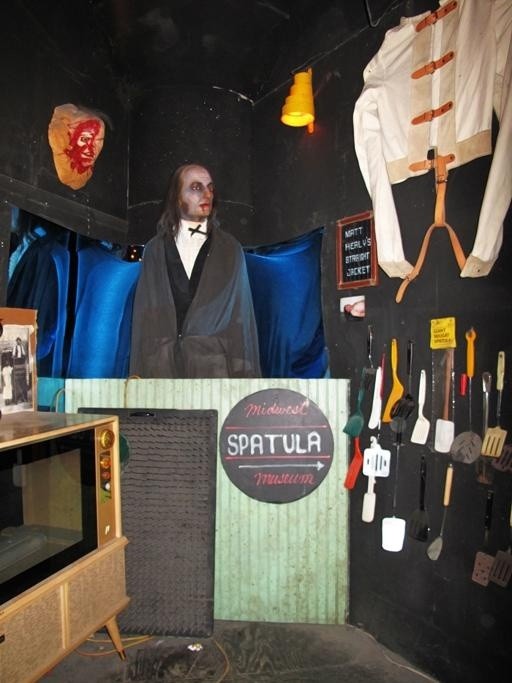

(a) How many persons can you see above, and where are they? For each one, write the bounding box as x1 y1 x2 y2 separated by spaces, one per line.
2 337 28 371
129 163 261 377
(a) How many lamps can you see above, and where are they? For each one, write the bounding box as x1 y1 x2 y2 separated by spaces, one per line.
281 66 316 133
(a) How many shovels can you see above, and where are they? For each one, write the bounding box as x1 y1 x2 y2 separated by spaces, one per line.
343 325 512 588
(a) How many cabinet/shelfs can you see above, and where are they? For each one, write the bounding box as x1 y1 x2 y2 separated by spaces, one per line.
0 412 132 683
